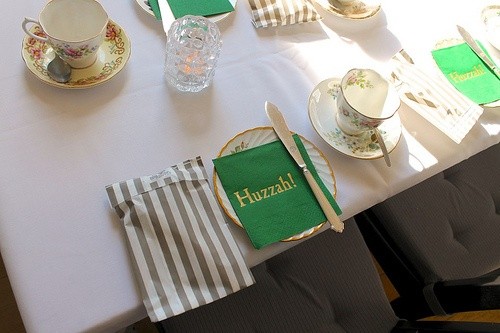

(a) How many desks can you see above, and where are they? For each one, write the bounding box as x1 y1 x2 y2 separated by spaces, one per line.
0 0 500 333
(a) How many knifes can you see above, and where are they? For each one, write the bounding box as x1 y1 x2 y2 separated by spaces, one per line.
157 0 178 39
457 25 500 81
265 99 345 234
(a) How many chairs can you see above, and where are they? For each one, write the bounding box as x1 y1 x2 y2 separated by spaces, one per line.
153 144 499 332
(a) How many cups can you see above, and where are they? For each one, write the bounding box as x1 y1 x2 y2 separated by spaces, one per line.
338 69 400 137
21 0 109 69
162 14 222 93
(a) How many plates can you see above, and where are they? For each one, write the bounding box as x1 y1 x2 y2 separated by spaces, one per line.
212 127 337 241
479 3 500 49
307 79 402 159
135 0 238 26
22 14 132 90
317 0 383 20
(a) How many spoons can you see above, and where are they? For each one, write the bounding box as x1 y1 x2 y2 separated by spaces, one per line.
46 51 71 83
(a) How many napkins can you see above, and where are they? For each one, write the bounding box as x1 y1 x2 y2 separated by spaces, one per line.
431 44 498 105
211 136 344 250
151 0 233 21
